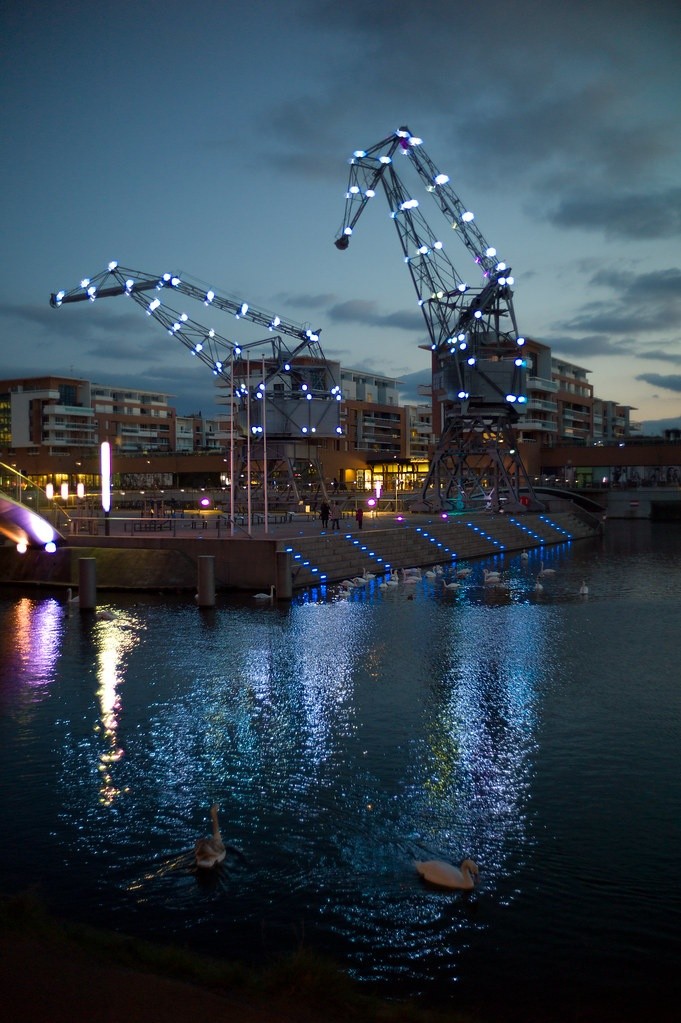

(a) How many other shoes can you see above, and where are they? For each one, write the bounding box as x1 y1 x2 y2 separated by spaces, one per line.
337 528 340 529
332 528 334 529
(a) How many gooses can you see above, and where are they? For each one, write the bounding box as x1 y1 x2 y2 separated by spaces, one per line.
415 860 480 890
194 804 226 868
66 547 590 604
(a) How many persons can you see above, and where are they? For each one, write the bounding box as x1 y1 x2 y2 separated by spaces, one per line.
331 502 342 530
333 478 339 494
409 480 412 488
320 499 331 528
356 509 363 529
